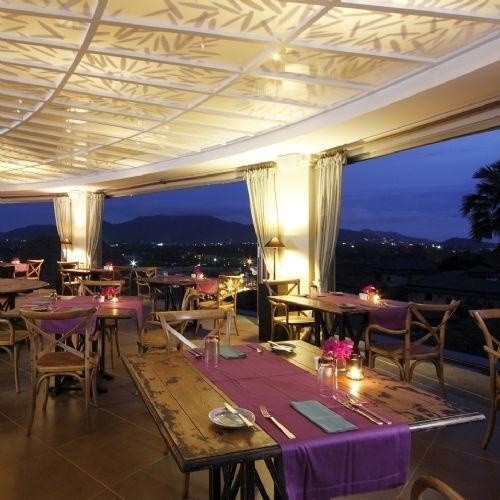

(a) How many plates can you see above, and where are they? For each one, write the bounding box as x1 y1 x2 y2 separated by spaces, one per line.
272 343 296 352
30 304 47 312
208 406 256 428
336 303 356 308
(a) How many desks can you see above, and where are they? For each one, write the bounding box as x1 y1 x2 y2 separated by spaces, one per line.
266 290 413 369
133 273 219 312
3 291 145 397
118 340 487 499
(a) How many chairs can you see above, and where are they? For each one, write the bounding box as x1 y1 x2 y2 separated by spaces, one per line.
2 256 126 436
467 308 500 452
364 299 462 387
262 278 318 344
193 274 244 335
131 264 157 300
153 308 237 351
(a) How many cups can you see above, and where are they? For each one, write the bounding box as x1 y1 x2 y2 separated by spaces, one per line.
196 270 204 280
163 271 169 278
191 274 197 279
310 285 318 301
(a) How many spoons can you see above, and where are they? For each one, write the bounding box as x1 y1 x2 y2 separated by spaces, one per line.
347 392 393 426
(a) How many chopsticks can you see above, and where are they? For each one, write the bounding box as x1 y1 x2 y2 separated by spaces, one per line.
223 401 254 429
267 340 292 353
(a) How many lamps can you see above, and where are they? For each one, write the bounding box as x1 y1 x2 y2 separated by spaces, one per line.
62 239 72 261
265 236 285 280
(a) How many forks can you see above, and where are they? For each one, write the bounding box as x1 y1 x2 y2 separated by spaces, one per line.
259 406 297 442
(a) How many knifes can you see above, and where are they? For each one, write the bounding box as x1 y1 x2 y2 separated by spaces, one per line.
333 395 383 426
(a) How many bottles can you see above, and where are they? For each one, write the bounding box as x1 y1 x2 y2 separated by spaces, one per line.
315 357 337 398
350 353 363 380
204 334 220 370
47 289 59 311
90 295 105 314
376 297 382 309
